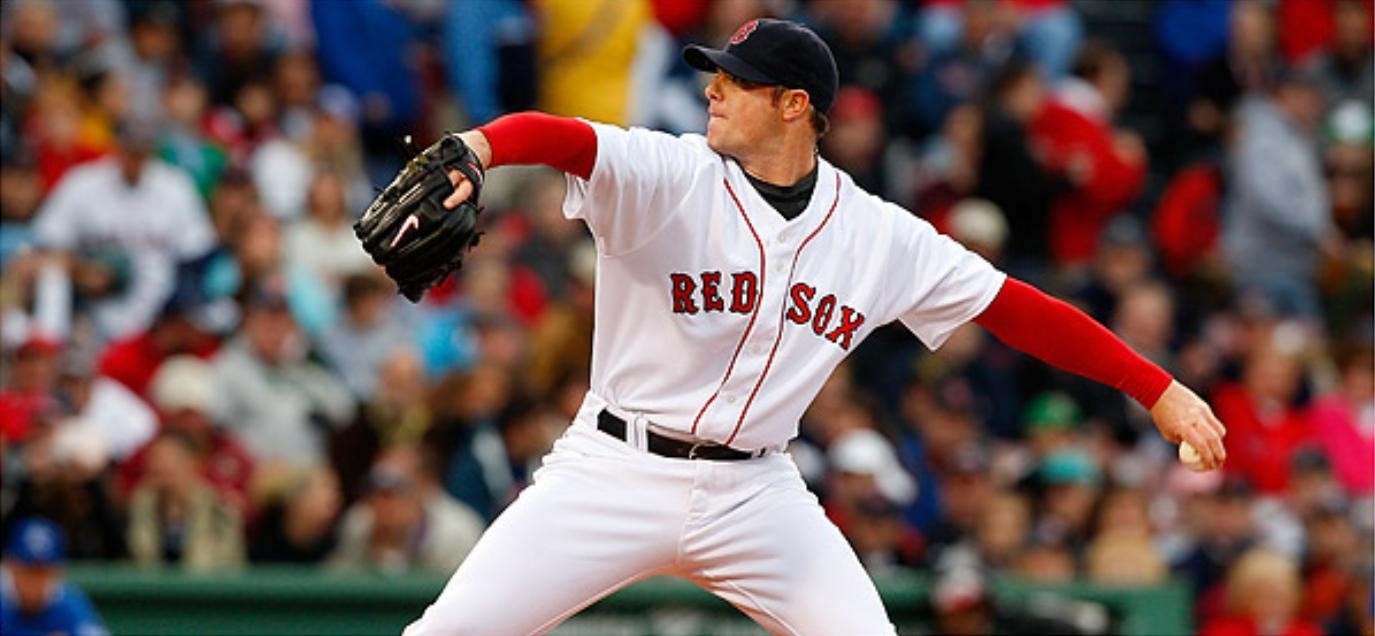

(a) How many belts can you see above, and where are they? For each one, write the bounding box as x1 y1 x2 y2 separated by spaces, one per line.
598 410 760 462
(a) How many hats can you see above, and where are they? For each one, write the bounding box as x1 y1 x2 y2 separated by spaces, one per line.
17 524 60 563
679 16 838 119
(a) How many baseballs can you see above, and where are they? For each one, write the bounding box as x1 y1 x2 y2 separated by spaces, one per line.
1179 441 1207 472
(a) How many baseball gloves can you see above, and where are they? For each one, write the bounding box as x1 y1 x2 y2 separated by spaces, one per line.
353 131 486 305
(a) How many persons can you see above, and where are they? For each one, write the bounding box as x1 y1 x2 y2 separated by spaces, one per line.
0 0 1375 636
351 18 1226 636
0 520 113 636
